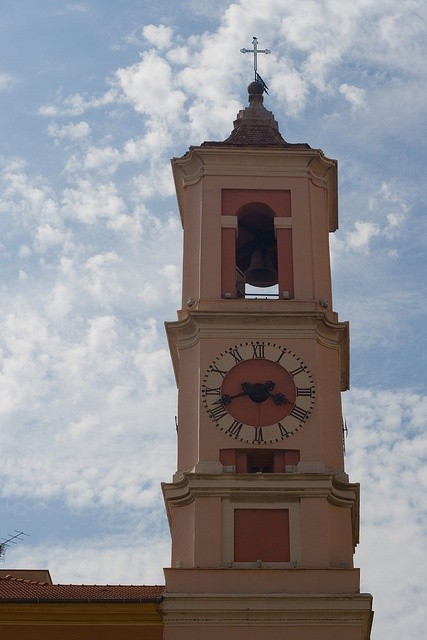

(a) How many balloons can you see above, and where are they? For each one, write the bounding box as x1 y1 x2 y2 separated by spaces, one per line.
202 338 316 446
